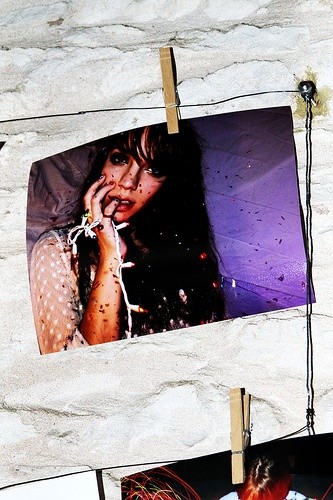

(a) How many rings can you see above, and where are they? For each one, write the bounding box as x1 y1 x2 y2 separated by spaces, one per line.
84 209 93 219
102 214 113 218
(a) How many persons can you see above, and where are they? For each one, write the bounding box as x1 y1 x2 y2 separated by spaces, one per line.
29 120 230 356
216 451 318 500
